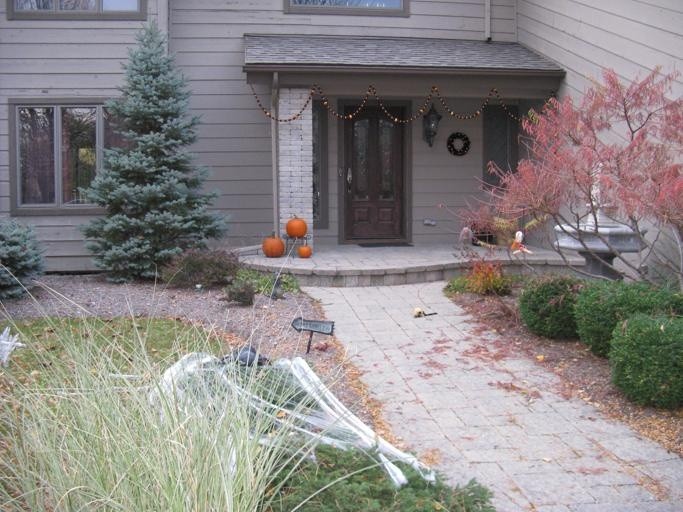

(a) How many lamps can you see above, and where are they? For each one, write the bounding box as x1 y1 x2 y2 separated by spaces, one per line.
422 102 443 146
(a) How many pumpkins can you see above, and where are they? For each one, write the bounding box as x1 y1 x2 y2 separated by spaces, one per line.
298 246 312 257
262 231 285 257
287 215 307 238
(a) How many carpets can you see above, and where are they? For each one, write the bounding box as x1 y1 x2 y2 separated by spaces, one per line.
358 242 414 248
